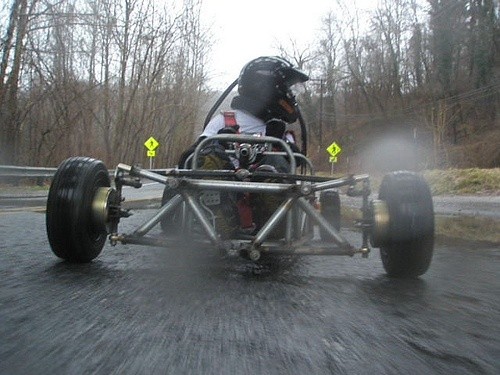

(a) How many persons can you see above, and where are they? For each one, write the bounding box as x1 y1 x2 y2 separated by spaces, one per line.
179 55 311 240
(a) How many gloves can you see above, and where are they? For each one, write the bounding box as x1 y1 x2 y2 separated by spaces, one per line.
218 125 241 146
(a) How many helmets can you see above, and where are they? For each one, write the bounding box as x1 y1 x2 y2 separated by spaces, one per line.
238 56 308 116
